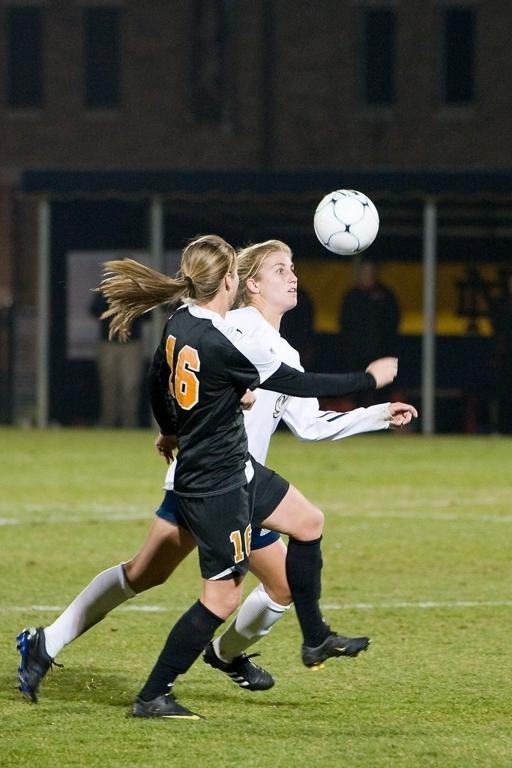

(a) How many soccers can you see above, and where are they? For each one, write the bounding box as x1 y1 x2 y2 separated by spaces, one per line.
312 185 380 256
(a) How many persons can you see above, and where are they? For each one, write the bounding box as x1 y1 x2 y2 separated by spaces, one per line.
11 237 419 705
86 255 153 430
95 234 400 721
337 258 402 435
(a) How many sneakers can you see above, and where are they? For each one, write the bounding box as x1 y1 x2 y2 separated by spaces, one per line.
132 690 206 720
201 639 274 690
16 626 64 702
302 632 372 671
